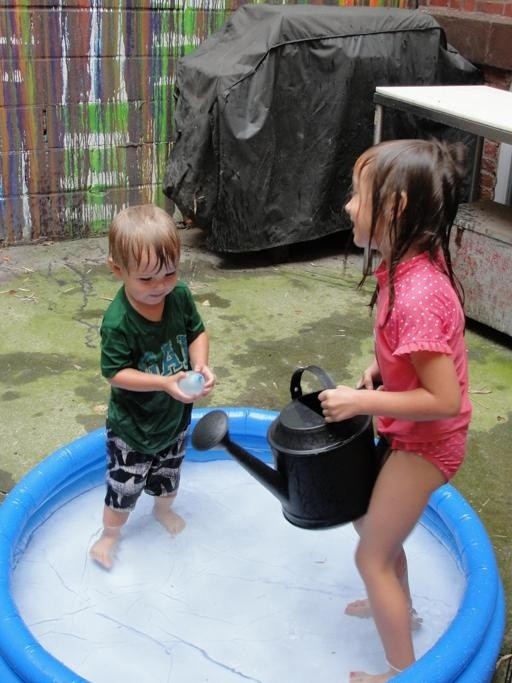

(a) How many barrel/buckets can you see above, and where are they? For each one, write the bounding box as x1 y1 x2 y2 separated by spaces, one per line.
192 363 399 531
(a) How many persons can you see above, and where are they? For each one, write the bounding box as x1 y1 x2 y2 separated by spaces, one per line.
88 203 218 573
316 138 471 682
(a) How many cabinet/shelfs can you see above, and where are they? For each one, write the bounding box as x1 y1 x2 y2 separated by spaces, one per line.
362 84 512 338
